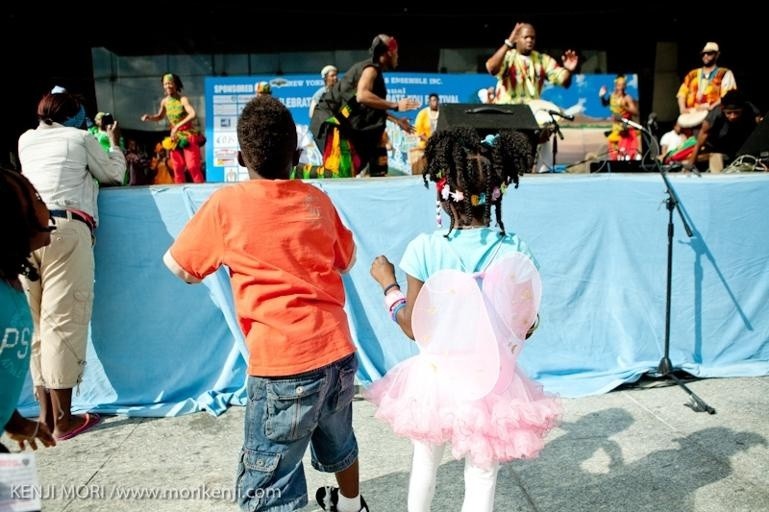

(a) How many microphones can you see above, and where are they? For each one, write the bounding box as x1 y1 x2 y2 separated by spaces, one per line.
647 112 657 126
615 115 651 135
549 109 574 120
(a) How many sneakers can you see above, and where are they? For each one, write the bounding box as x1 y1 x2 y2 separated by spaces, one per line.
315 484 369 512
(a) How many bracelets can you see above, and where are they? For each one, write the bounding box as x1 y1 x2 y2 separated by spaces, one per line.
384 281 407 322
527 313 540 334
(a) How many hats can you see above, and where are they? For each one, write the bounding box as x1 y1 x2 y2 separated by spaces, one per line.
701 41 720 53
321 64 338 79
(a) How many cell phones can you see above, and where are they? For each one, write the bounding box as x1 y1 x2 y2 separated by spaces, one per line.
103 113 113 125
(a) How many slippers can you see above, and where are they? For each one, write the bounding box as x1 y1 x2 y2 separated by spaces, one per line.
51 411 101 442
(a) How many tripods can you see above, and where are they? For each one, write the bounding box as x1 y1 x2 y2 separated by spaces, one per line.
617 131 716 414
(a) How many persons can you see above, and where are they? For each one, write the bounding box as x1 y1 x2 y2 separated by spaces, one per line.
370 126 568 512
0 169 55 454
162 95 369 512
12 21 765 185
16 85 127 442
